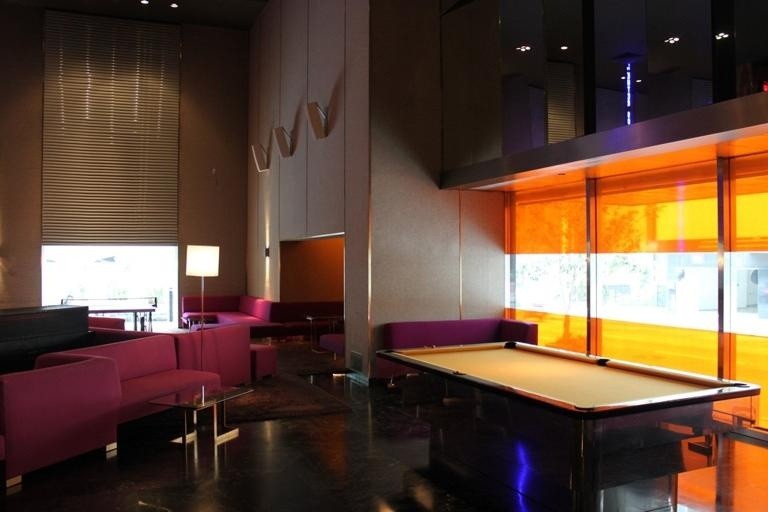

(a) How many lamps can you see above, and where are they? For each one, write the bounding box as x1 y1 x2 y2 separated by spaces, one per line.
185 244 220 334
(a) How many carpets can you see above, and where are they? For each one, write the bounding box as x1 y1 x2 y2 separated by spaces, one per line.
203 371 353 423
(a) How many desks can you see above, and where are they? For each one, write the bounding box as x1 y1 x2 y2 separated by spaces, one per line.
61 297 157 333
376 339 760 461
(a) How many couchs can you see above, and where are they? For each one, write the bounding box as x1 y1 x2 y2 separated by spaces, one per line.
383 319 537 389
0 352 123 487
63 334 221 425
271 302 344 342
88 295 277 387
320 333 345 361
